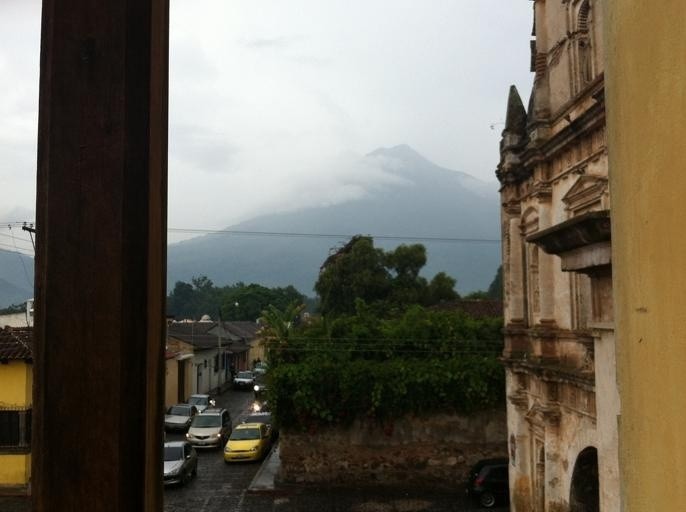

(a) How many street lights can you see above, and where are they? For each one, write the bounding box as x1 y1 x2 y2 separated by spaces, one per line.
219 300 240 383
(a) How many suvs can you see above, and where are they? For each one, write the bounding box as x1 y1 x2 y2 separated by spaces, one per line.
462 457 508 510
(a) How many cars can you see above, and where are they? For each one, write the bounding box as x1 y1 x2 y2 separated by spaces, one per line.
164 360 279 488
